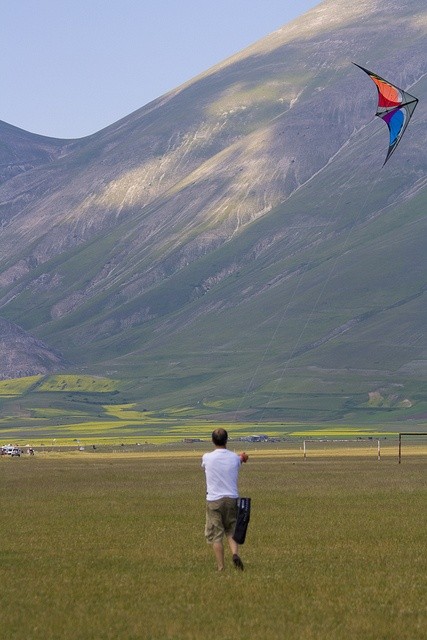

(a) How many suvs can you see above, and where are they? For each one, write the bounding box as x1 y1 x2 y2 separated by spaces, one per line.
11 448 20 456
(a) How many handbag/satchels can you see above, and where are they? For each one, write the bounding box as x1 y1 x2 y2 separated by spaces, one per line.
233 498 250 544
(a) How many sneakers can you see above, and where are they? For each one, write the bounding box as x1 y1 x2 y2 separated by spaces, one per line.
233 554 243 571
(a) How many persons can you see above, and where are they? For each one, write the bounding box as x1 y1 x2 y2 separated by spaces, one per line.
201 428 248 573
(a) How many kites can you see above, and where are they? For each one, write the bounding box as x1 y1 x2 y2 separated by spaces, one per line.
353 61 419 167
